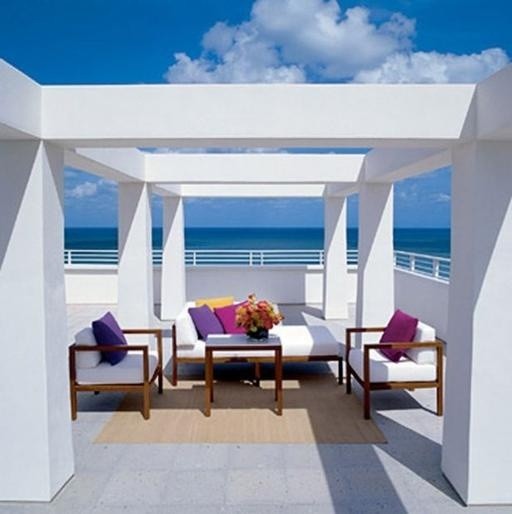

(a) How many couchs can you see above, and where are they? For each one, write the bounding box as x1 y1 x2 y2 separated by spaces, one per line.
172 301 344 384
345 323 444 419
68 327 163 420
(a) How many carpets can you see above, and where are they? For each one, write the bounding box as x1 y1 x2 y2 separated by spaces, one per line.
92 372 386 445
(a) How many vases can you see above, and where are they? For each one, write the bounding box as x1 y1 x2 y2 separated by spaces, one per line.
247 326 269 339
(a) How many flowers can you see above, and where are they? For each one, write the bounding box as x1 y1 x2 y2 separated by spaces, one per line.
235 291 284 332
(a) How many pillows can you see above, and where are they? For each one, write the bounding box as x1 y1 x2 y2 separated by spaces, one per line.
214 301 248 334
195 296 234 313
188 304 224 340
91 311 127 366
379 309 418 363
